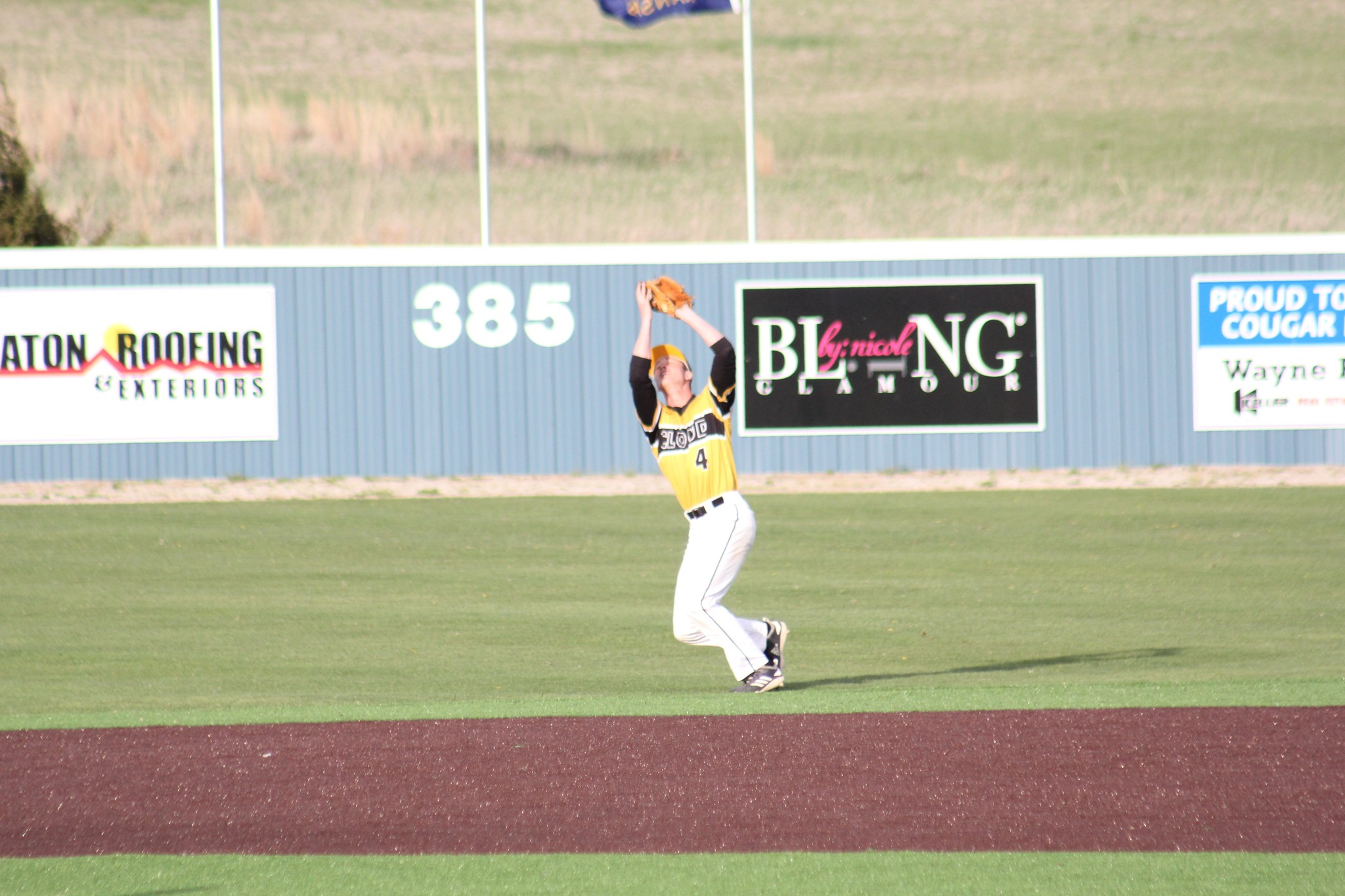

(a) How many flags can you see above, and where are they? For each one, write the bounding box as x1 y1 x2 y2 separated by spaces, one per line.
595 0 743 29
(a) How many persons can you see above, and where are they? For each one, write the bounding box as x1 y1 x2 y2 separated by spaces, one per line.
628 281 789 694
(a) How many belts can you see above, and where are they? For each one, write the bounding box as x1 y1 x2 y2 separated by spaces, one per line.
688 497 724 520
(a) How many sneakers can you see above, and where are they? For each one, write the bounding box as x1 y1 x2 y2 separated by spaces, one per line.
728 617 787 693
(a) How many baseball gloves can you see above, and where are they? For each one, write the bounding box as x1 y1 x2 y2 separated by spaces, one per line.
646 276 692 320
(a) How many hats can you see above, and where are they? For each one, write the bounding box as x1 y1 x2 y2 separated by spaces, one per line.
648 344 689 379
